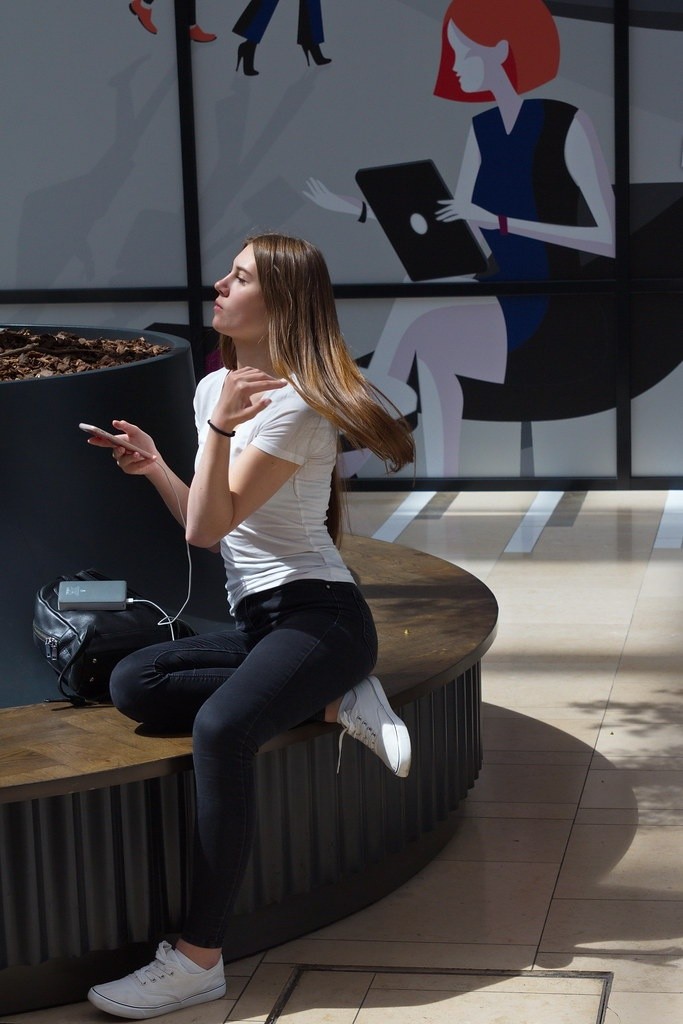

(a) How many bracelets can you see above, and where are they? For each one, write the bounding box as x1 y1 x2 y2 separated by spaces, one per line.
207 420 236 437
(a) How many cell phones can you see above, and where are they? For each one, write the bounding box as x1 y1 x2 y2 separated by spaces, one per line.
79 423 153 460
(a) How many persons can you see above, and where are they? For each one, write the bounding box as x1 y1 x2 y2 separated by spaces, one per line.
87 234 413 1020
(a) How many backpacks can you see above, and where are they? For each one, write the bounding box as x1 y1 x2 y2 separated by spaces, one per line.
31 569 197 708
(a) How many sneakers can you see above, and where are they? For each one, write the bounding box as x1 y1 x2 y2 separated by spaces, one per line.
336 675 410 778
86 940 228 1019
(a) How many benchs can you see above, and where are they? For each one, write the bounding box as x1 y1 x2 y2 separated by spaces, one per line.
0 531 499 1017
